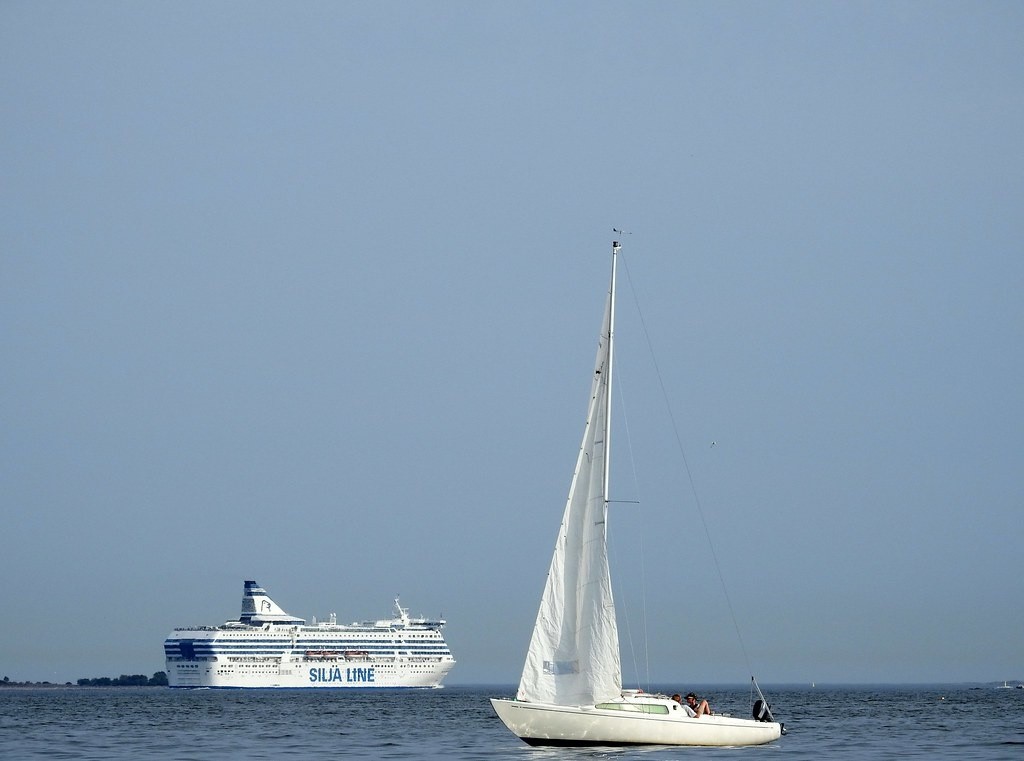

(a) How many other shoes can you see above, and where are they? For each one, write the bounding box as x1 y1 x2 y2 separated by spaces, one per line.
709 711 715 716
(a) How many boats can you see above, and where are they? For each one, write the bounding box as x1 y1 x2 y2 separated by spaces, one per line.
162 579 459 691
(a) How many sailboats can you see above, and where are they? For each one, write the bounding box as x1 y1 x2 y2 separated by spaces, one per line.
487 223 788 748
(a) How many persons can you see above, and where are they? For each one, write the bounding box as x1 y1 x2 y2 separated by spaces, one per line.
684 693 699 713
672 694 710 718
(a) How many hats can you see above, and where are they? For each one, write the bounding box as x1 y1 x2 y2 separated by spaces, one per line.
684 692 698 700
